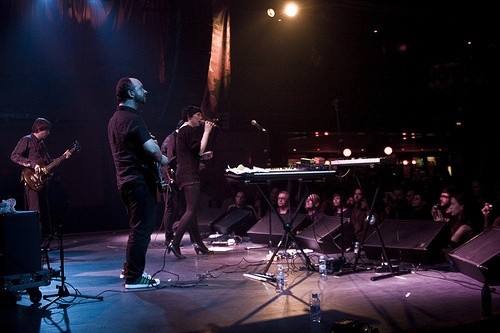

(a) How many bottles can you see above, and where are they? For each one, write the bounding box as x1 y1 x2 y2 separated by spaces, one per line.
319 256 327 277
276 265 284 287
310 292 321 322
354 242 359 254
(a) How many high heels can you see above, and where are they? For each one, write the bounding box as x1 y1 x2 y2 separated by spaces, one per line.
167 242 186 259
194 244 215 255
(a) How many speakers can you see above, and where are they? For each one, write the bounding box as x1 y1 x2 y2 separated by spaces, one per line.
247 212 313 246
448 226 500 287
362 219 452 264
197 209 224 234
212 209 256 236
294 216 357 254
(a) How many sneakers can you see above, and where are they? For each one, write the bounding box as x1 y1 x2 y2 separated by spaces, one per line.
120 268 151 280
124 273 160 288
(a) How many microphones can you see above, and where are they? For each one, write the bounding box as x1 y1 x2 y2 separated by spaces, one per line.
200 120 217 127
251 120 267 135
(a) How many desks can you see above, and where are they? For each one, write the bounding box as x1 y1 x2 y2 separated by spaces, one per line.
225 174 340 275
334 157 412 281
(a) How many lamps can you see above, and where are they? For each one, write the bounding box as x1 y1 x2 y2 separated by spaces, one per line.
267 4 280 17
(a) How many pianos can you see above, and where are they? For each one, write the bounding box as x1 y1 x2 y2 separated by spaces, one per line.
225 156 411 283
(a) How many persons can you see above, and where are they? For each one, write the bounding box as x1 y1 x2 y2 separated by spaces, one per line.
11 118 71 251
166 105 213 258
108 77 168 288
383 181 433 220
274 190 299 214
228 192 259 220
431 186 475 271
306 194 321 215
332 186 373 242
160 121 196 247
465 178 496 233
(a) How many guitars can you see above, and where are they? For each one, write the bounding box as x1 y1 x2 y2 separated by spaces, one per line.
23 140 82 192
149 134 166 193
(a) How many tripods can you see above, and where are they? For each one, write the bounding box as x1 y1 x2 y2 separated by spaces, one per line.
39 183 103 310
